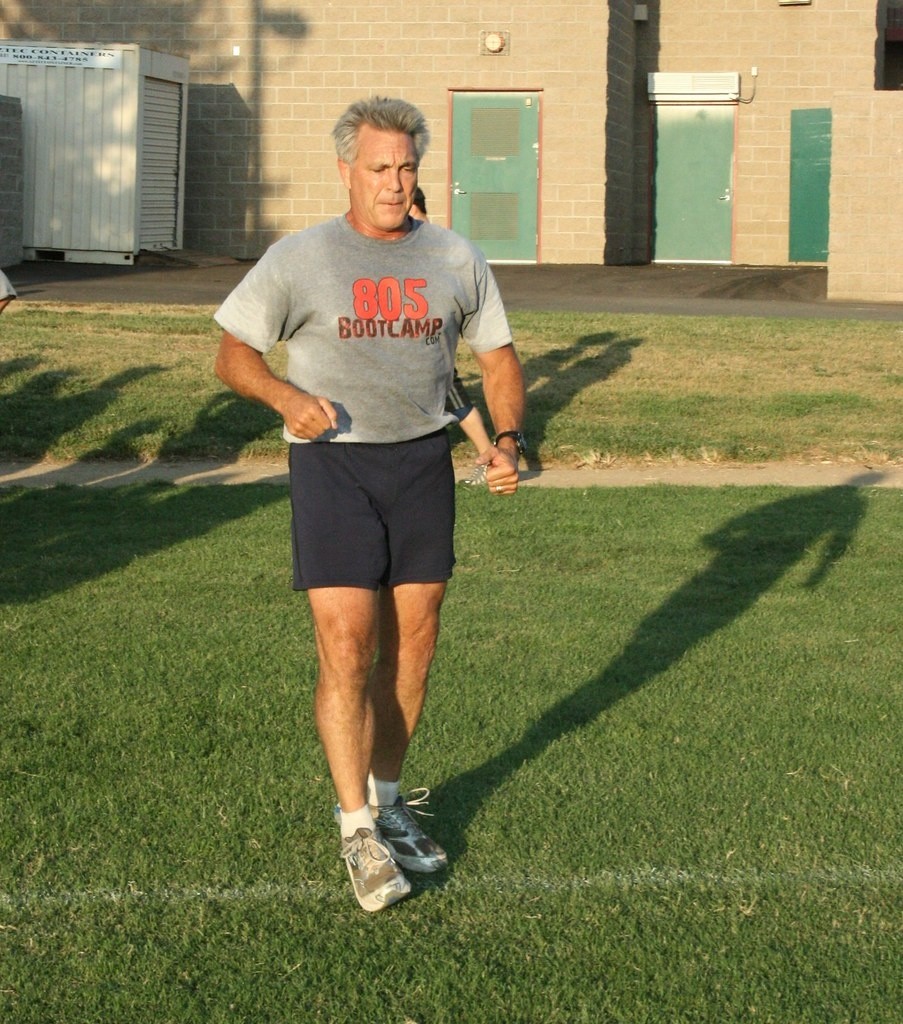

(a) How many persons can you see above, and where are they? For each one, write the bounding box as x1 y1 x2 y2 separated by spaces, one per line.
213 98 527 913
407 186 492 456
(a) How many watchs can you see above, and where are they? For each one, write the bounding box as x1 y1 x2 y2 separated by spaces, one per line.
493 429 527 452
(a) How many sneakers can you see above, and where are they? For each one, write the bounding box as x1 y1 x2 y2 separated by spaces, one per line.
341 823 411 914
465 461 490 485
333 787 449 874
